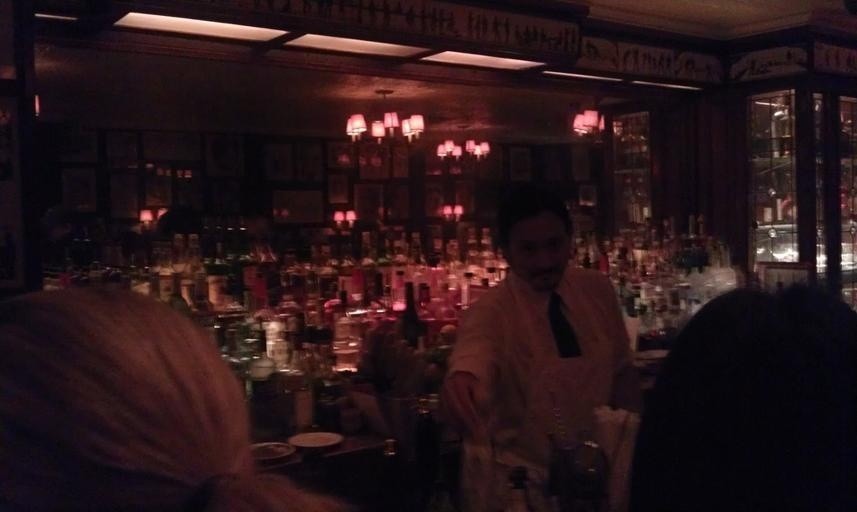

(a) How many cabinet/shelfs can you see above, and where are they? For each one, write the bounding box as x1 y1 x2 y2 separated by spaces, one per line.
613 166 649 233
753 154 856 233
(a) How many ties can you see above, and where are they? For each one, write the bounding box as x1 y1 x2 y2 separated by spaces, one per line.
546 296 580 360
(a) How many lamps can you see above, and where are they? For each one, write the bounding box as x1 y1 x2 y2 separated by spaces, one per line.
331 87 605 230
139 209 155 229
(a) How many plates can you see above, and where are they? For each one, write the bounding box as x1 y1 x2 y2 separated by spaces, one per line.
288 431 343 453
248 440 297 461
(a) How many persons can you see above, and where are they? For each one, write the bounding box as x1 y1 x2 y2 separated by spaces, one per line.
434 188 638 436
0 281 347 507
628 277 855 511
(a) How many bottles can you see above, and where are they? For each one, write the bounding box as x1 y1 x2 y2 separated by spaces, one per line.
607 111 658 224
43 209 735 417
290 384 316 431
755 98 855 223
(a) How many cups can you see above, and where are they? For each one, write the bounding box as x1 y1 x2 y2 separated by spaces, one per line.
766 230 855 271
841 285 855 311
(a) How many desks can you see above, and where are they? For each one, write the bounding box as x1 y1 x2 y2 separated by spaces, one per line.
254 429 382 470
